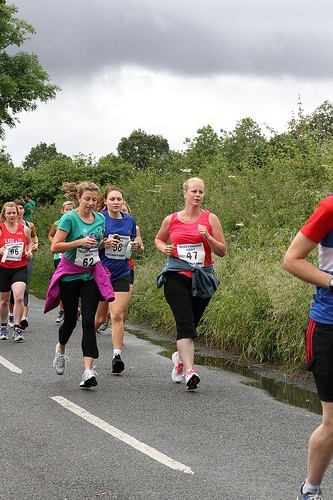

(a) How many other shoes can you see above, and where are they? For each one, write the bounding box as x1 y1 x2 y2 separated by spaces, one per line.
97 323 109 333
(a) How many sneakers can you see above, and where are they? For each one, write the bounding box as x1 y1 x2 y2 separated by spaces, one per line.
0 325 8 340
171 351 184 383
111 354 124 373
12 326 24 341
7 313 14 328
20 316 28 330
52 343 70 375
297 481 323 500
185 367 200 391
77 308 81 321
55 310 64 325
80 366 99 388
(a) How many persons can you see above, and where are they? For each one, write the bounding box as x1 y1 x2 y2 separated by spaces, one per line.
0 202 34 341
48 201 81 324
283 193 333 500
95 186 144 373
99 201 144 345
44 180 115 388
155 177 226 390
8 199 38 330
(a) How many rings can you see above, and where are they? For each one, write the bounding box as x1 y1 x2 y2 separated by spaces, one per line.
90 242 92 244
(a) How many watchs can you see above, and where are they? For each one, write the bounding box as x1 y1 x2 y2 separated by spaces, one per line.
329 278 333 289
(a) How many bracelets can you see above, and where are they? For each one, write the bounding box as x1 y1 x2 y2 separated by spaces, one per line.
34 242 38 244
